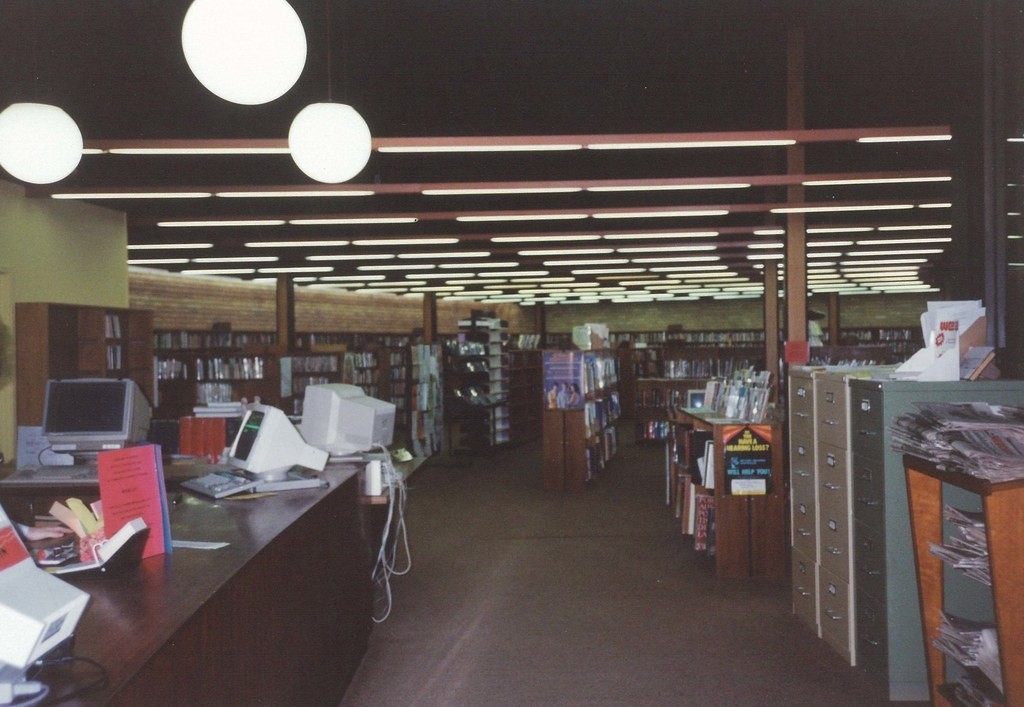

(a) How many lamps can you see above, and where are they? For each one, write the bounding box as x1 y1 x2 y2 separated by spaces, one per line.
288 0 372 186
0 0 84 186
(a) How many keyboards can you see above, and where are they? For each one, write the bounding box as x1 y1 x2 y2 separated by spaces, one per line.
180 472 265 499
0 464 99 486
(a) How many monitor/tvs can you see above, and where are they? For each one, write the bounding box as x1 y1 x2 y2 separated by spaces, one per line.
301 383 396 462
0 506 90 704
228 403 330 493
42 379 152 464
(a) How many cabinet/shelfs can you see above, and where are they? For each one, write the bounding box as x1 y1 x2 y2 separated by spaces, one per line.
14 303 539 449
541 323 1024 707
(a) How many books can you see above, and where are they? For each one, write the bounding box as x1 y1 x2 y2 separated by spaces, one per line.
105 314 122 370
155 316 1023 707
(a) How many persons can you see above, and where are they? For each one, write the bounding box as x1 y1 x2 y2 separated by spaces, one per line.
568 383 581 407
10 521 73 542
557 382 569 408
548 384 558 408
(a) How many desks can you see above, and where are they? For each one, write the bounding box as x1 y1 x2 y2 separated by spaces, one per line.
360 456 428 583
